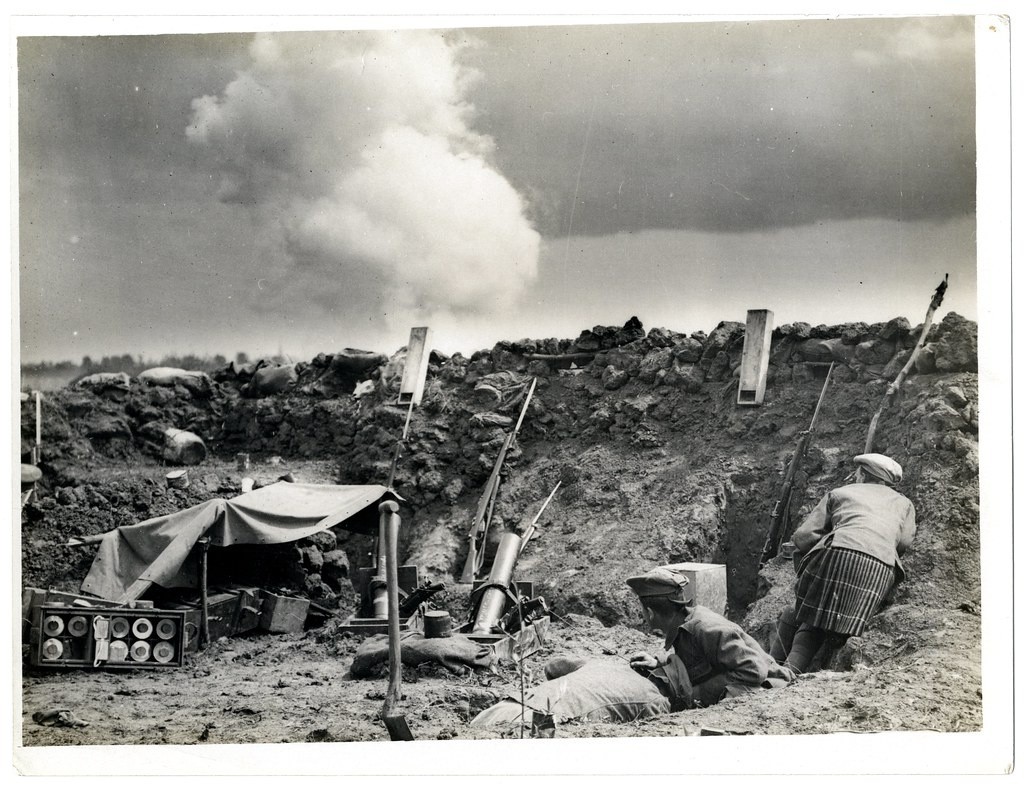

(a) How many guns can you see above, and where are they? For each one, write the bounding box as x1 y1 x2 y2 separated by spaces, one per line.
368 391 416 568
459 376 537 584
755 360 835 575
519 480 563 556
28 393 41 503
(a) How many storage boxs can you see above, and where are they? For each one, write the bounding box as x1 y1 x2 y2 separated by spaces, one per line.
262 591 310 634
660 563 728 618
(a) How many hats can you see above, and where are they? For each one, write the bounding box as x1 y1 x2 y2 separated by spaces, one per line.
853 452 902 484
627 567 689 596
662 654 693 708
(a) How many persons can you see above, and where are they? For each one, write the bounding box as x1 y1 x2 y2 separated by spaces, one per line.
768 452 917 674
469 565 797 724
